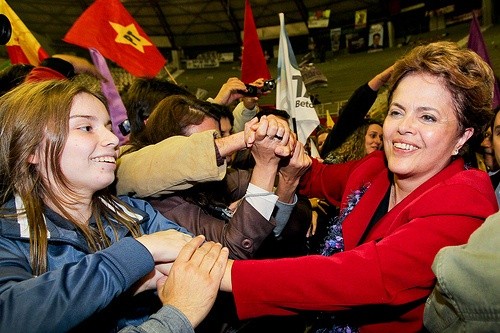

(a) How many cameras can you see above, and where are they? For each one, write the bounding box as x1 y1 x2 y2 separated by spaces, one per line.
237 84 257 97
260 79 276 92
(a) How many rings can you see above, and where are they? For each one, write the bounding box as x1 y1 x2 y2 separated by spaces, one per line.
274 134 283 140
304 151 308 155
265 134 274 139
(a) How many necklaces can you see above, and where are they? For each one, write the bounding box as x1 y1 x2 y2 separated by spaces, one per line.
392 182 398 205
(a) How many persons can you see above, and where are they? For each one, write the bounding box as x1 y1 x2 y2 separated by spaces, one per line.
312 59 398 256
132 41 500 333
105 76 298 199
298 35 320 68
358 14 365 23
0 78 206 333
139 95 312 262
206 78 332 168
368 33 383 51
118 235 229 333
423 106 500 333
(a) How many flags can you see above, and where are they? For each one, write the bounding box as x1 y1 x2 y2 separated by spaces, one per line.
241 0 273 96
63 0 168 78
0 0 52 67
467 11 500 110
275 12 320 147
